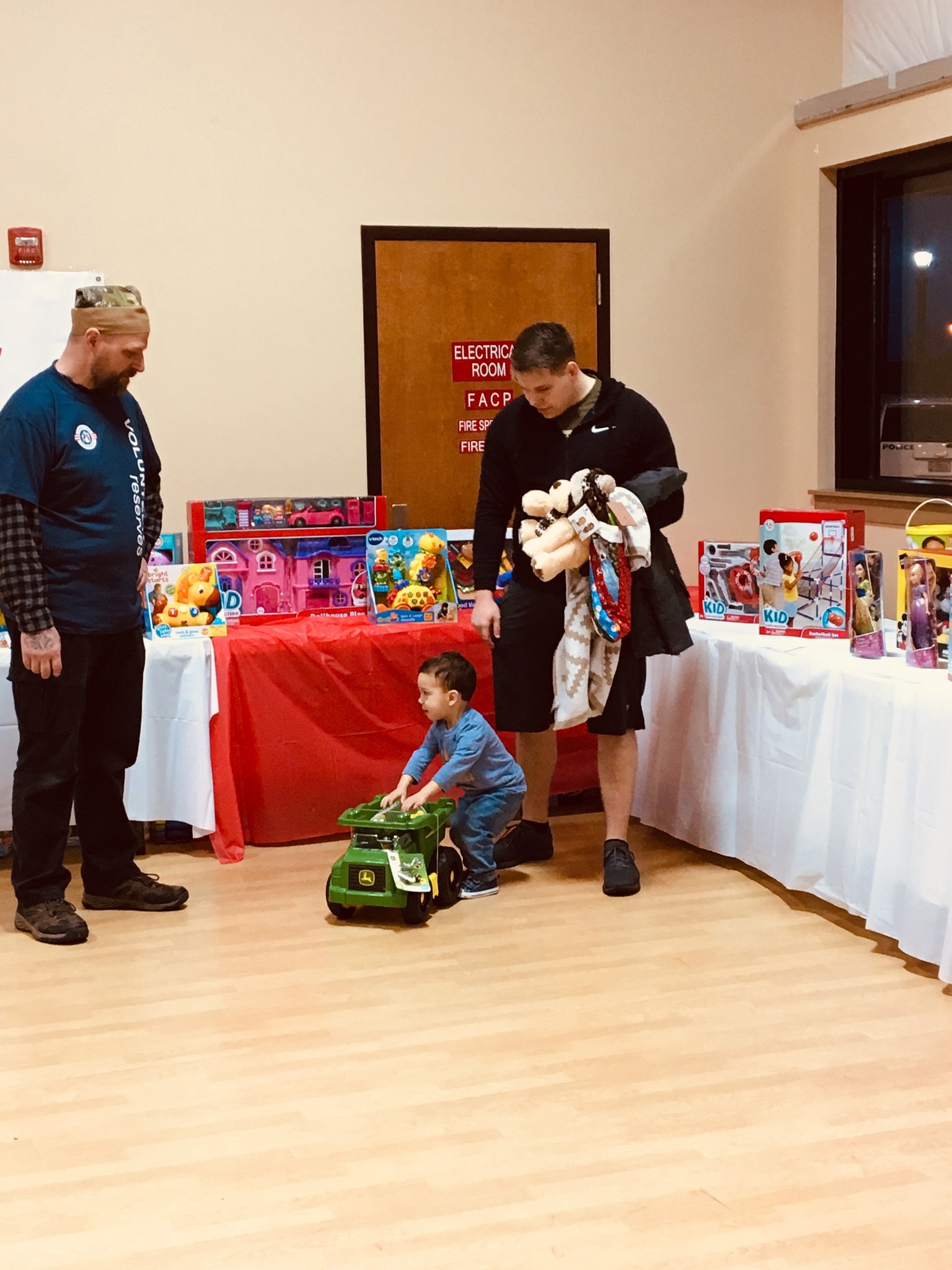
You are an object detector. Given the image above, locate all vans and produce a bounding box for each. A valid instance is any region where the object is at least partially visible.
[879,394,952,498]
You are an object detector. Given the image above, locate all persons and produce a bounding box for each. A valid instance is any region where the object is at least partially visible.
[148,583,162,606]
[470,321,695,897]
[908,564,932,649]
[854,559,873,632]
[0,285,190,945]
[760,539,804,628]
[380,651,527,898]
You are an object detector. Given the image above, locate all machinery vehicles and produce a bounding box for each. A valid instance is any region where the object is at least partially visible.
[325,792,466,925]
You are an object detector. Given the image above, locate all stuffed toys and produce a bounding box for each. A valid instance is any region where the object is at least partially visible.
[518,469,616,583]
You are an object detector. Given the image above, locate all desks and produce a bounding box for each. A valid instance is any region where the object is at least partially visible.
[0,635,219,854]
[208,608,599,864]
[629,612,951,990]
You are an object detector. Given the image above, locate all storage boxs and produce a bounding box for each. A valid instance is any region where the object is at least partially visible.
[365,530,459,626]
[143,563,228,641]
[698,541,759,623]
[759,508,865,639]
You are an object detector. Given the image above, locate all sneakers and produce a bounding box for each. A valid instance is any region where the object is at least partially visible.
[492,819,554,869]
[603,839,640,897]
[82,870,189,912]
[14,898,89,945]
[457,871,500,899]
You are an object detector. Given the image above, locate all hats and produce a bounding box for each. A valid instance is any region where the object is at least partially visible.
[71,285,150,335]
